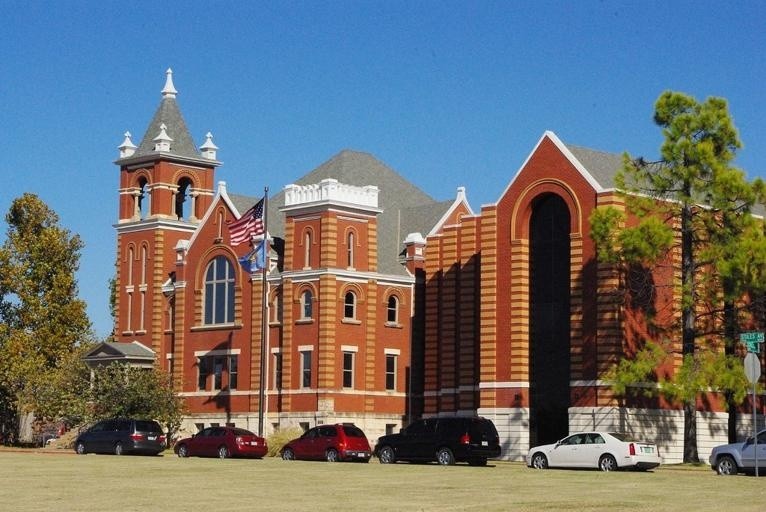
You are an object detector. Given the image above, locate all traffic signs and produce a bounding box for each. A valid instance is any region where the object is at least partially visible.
[739,332,765,342]
[747,341,761,353]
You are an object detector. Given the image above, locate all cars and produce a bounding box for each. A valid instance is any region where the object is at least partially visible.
[524,431,661,472]
[708,428,766,476]
[280,424,372,464]
[173,427,269,459]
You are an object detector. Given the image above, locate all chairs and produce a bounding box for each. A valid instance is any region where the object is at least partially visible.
[580,438,585,443]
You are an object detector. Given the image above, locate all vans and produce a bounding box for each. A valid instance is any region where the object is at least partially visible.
[73,417,167,456]
[373,416,502,466]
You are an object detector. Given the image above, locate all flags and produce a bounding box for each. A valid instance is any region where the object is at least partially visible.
[238,240,267,273]
[226,198,266,246]
